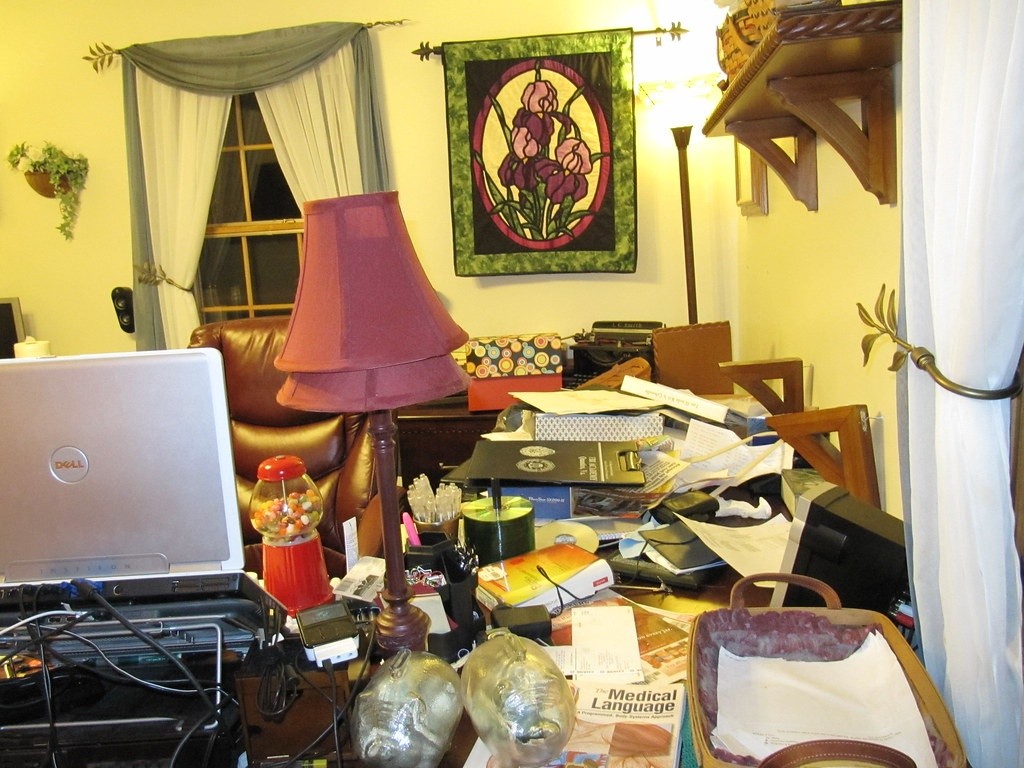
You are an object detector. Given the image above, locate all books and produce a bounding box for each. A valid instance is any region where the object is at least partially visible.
[537,681,686,768]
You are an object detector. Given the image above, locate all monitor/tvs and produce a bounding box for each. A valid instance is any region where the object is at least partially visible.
[0,297,26,361]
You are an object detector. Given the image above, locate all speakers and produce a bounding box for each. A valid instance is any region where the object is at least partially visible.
[112,287,135,334]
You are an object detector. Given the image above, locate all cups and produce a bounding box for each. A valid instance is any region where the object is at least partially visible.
[412,512,463,547]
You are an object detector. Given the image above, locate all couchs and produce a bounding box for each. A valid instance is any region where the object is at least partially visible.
[188,315,378,555]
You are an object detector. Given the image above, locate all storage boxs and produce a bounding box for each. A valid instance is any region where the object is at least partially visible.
[653,320,734,395]
[465,333,562,379]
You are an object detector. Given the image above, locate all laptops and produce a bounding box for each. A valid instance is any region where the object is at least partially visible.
[0,346,301,639]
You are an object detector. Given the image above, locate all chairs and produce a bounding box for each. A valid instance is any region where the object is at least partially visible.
[241,544,351,581]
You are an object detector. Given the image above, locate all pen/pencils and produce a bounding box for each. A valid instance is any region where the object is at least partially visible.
[404,471,465,524]
[401,513,421,548]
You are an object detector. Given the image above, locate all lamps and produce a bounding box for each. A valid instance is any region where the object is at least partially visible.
[273,193,470,647]
[651,90,711,324]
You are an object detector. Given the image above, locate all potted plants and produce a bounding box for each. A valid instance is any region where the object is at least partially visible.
[4,141,89,241]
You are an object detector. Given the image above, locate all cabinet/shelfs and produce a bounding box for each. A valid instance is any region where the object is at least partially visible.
[397,399,502,524]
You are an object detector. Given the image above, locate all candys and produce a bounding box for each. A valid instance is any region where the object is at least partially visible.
[250,489,320,541]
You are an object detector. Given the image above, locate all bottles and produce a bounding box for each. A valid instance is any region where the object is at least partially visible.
[248,454,336,617]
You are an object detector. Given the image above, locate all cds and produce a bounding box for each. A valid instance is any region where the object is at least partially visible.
[462,496,535,567]
[535,521,598,555]
